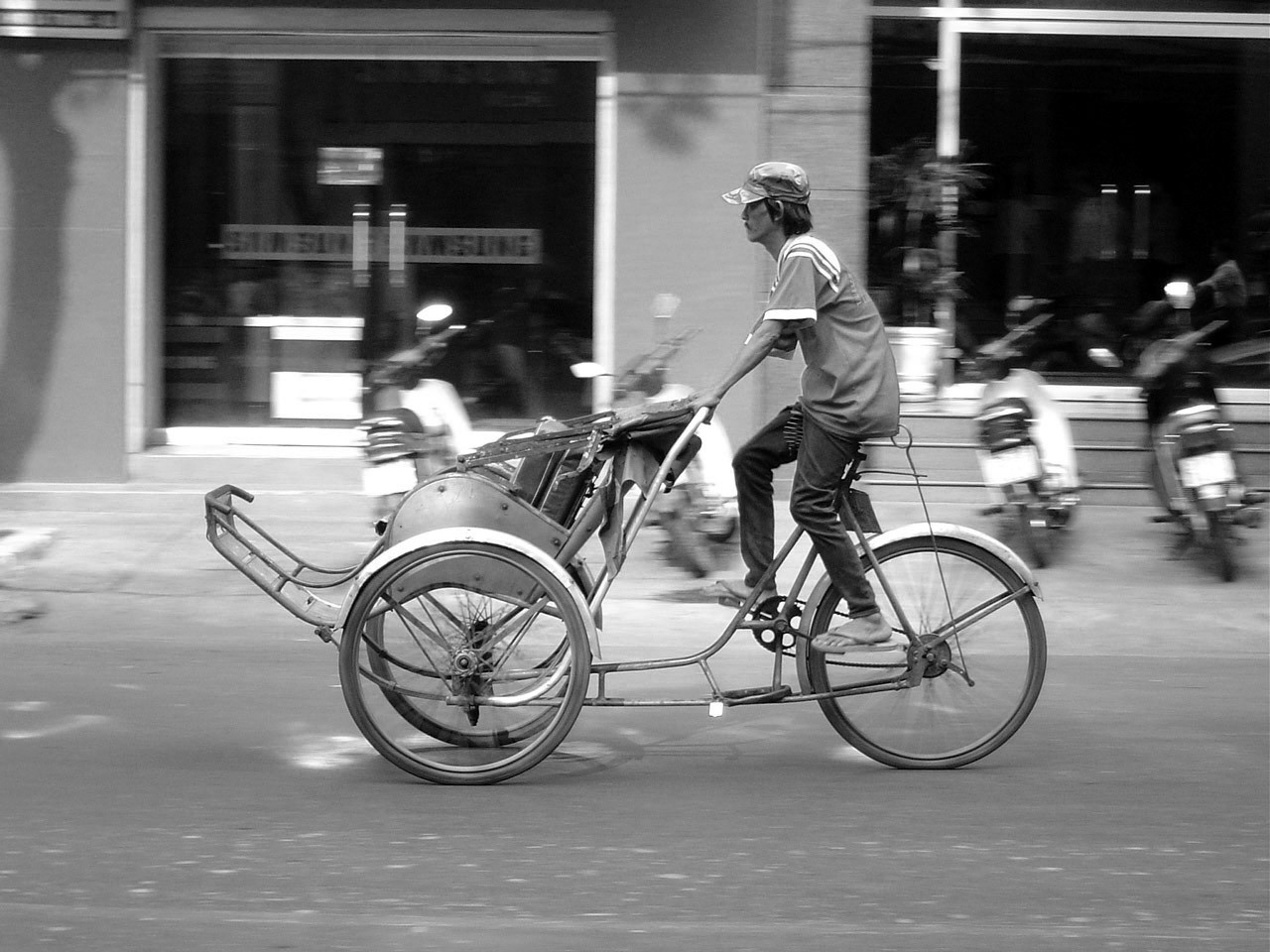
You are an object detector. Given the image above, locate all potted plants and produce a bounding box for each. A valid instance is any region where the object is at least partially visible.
[857,135,994,404]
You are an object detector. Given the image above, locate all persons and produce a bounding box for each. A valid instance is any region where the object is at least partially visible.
[683,160,903,651]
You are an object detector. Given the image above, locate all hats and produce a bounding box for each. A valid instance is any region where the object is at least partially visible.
[721,159,810,205]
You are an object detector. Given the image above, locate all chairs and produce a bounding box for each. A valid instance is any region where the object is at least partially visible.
[453,415,596,529]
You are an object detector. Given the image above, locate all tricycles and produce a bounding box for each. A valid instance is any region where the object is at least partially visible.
[206,405,1049,785]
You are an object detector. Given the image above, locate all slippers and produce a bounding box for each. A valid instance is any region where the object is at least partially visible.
[700,577,764,609]
[809,626,900,652]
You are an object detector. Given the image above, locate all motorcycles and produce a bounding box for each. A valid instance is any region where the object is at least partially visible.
[355,301,477,539]
[1087,260,1268,583]
[971,296,1082,566]
[568,293,738,578]
